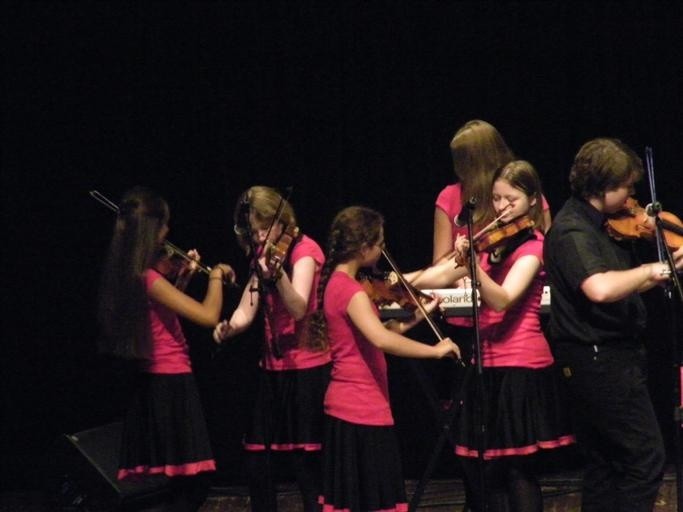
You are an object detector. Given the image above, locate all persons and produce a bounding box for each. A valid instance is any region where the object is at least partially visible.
[434,120,552,357]
[114,185,235,510]
[542,136,682,511]
[454,161,576,511]
[307,209,461,510]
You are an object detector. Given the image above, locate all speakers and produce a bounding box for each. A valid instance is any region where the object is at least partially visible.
[46,423,173,511]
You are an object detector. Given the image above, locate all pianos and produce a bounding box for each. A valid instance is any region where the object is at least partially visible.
[375,286,553,316]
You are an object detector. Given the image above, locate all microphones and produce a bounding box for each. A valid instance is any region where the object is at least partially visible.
[232,199,255,235]
[454,197,478,226]
[646,202,661,217]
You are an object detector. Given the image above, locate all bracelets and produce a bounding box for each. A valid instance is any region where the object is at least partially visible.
[208,276,223,281]
[212,265,224,276]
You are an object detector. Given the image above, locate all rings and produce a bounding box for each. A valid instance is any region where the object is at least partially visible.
[660,271,664,276]
[663,266,666,271]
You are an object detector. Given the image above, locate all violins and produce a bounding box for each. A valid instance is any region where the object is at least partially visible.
[356,265,442,313]
[145,251,210,278]
[474,215,536,253]
[604,196,681,253]
[265,223,300,278]
